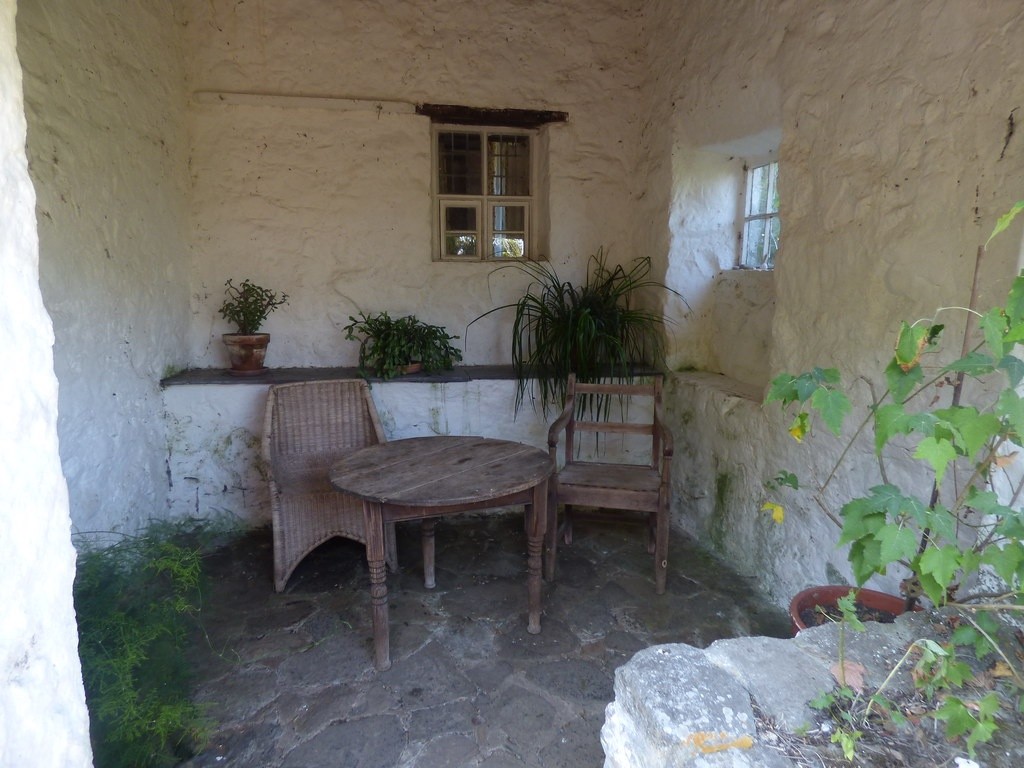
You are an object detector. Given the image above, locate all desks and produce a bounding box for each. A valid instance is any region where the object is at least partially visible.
[328,436,556,673]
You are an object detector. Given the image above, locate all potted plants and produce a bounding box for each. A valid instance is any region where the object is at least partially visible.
[217,277,291,371]
[340,310,463,384]
[461,241,696,460]
[756,199,1024,761]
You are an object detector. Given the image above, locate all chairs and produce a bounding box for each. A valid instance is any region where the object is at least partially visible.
[258,380,400,591]
[547,373,674,595]
